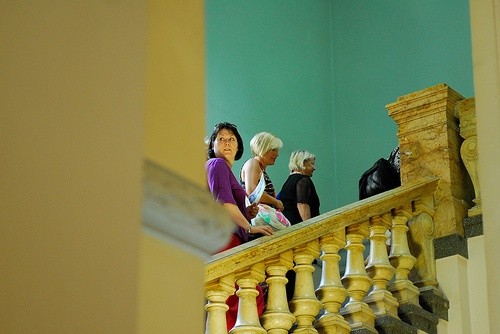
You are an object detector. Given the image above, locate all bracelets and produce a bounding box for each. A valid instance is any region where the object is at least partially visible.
[246,224,251,234]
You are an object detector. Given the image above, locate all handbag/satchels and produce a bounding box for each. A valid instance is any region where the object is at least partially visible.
[251,203,291,232]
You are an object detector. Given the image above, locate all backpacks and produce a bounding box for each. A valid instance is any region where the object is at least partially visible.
[359,147,401,200]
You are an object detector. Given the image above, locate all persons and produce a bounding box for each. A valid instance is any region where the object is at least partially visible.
[385,143,402,173]
[205,121,266,332]
[277,150,320,310]
[239,131,284,309]
